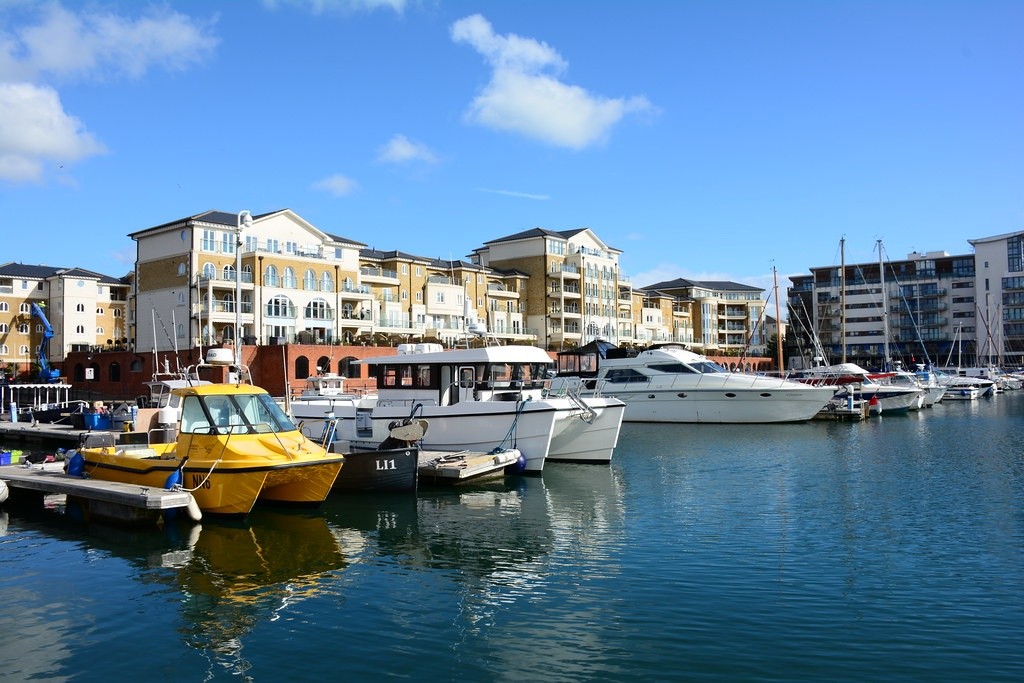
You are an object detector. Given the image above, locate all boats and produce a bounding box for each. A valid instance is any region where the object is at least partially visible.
[544,338,840,426]
[0,383,90,426]
[276,252,627,497]
[63,276,346,521]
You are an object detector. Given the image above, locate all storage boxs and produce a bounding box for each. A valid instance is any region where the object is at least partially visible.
[83,413,112,430]
[0,450,24,466]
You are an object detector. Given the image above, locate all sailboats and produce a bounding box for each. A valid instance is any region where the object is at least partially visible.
[734,234,1024,416]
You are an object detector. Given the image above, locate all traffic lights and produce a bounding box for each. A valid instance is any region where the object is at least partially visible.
[5,373,14,385]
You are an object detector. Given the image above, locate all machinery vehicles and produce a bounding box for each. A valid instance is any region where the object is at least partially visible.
[27,301,60,384]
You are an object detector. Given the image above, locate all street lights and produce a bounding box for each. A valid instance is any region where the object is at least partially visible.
[236,210,253,370]
